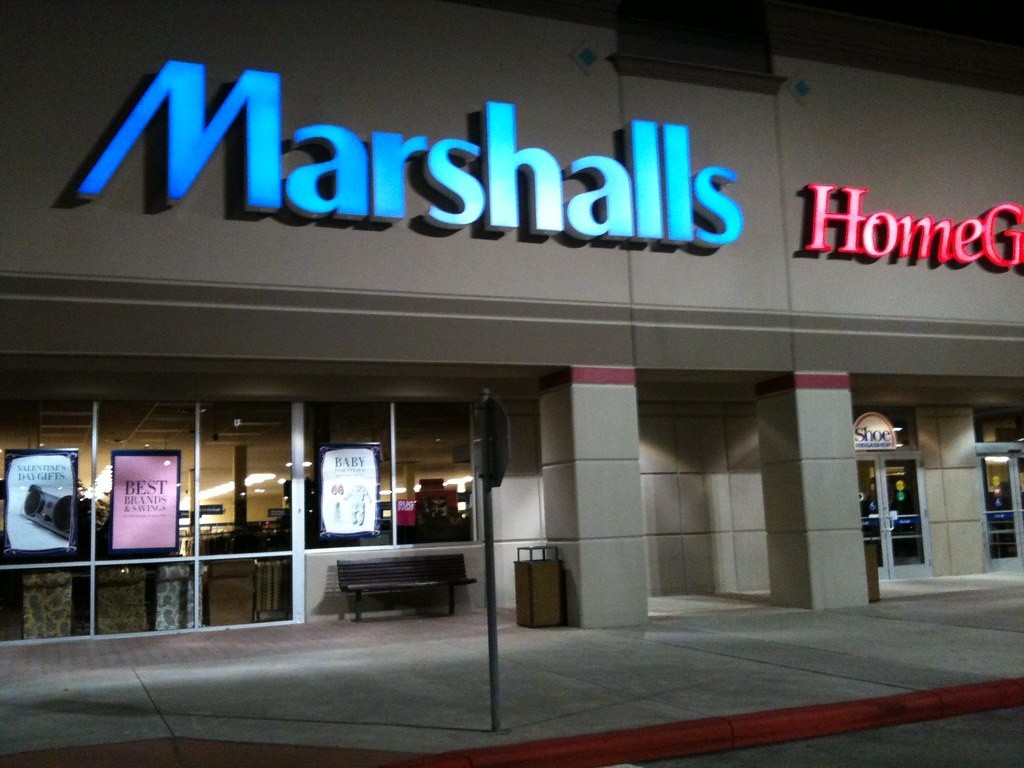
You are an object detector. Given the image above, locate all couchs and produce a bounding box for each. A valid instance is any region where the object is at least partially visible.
[252,558,292,623]
[154,560,210,630]
[95,566,151,635]
[20,570,76,639]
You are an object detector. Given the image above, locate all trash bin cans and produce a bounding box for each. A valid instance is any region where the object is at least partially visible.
[512,545,565,627]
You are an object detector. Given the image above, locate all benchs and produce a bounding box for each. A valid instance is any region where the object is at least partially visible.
[336,553,477,623]
